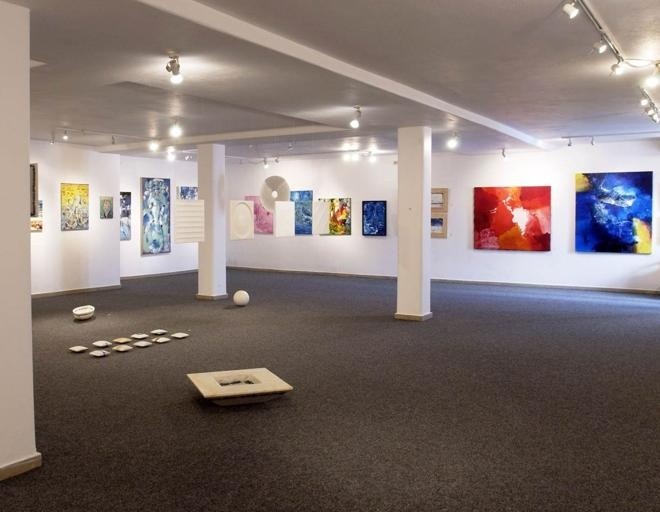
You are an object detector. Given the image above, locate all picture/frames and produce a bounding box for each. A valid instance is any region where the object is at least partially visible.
[431,213,448,238]
[431,188,448,212]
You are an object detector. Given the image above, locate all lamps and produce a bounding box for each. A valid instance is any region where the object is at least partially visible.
[179,149,280,167]
[638,81,660,125]
[560,0,629,80]
[349,104,363,129]
[46,127,162,152]
[164,48,184,85]
